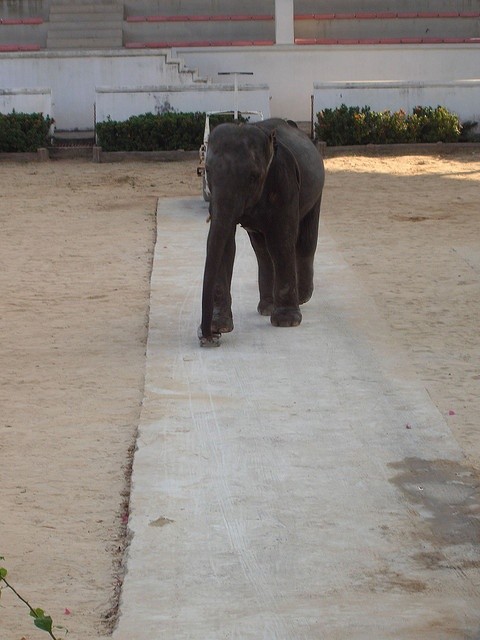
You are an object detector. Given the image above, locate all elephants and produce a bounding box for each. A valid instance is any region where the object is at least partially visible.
[196,115,326,348]
